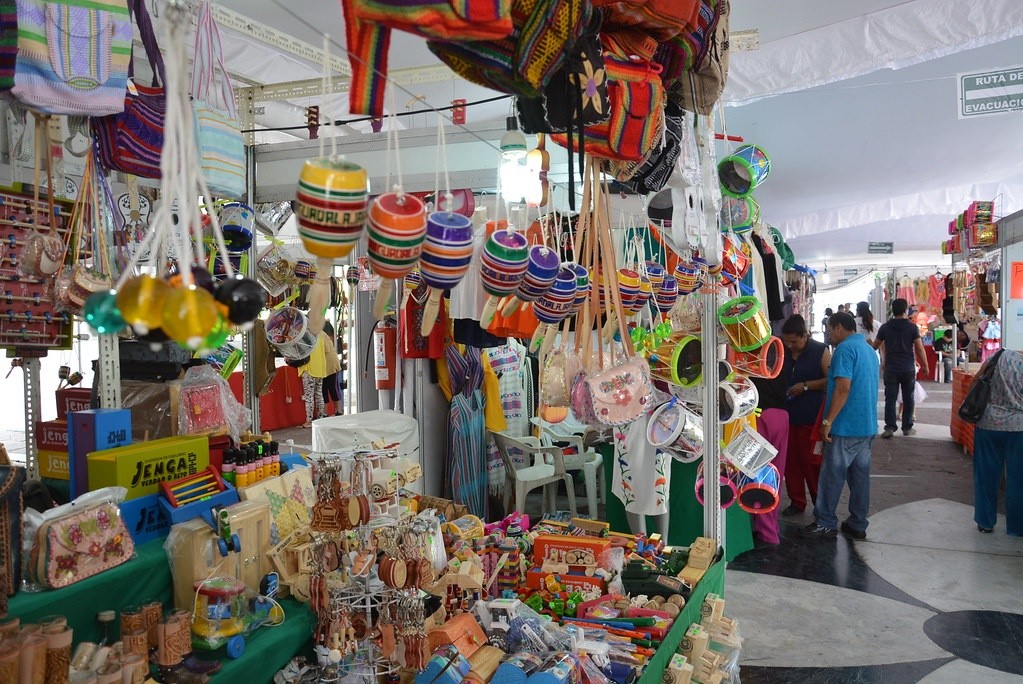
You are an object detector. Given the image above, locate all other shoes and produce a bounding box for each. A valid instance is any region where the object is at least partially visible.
[977,524,993,533]
[783,506,795,516]
[880,429,894,438]
[903,428,917,436]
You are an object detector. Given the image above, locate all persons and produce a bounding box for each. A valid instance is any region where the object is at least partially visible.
[933,329,962,382]
[798,312,880,538]
[968,348,1023,536]
[749,314,832,547]
[298,320,342,428]
[822,298,929,437]
[611,412,672,546]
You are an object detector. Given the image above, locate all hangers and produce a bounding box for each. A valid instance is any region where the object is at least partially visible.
[904,267,944,279]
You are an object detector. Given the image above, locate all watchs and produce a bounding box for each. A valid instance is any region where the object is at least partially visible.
[804,381,808,390]
[823,419,830,425]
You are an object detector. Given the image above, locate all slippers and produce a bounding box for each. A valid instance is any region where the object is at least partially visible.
[296,424,310,428]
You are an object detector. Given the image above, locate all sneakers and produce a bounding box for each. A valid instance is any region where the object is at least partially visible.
[841,520,867,539]
[798,522,838,537]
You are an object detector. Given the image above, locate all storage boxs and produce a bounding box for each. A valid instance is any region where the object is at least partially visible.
[35,340,235,547]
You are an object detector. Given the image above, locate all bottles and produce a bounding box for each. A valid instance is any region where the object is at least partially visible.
[785,367,796,400]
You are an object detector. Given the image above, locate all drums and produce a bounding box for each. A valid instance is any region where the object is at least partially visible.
[217,202,292,253]
[647,142,782,512]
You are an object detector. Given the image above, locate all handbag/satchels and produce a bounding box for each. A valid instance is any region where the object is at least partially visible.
[189,0,247,198]
[91,0,168,179]
[340,0,729,197]
[10,0,127,117]
[34,502,134,588]
[544,348,651,425]
[17,222,114,319]
[957,347,1005,424]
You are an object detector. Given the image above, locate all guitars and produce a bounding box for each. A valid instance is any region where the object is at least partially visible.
[434,98,478,218]
[524,131,550,207]
[116,171,158,268]
[291,104,321,212]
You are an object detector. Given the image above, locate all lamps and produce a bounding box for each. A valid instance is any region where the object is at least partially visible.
[820,254,833,285]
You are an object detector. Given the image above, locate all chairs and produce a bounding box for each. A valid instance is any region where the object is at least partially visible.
[483,430,580,522]
[528,416,609,521]
[933,347,965,383]
